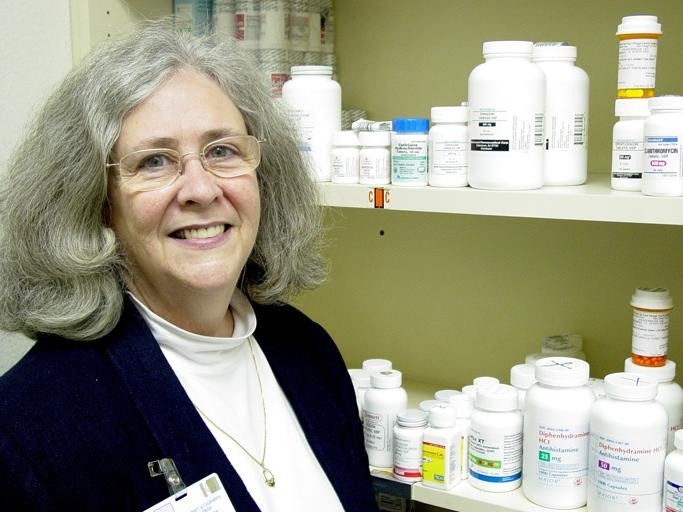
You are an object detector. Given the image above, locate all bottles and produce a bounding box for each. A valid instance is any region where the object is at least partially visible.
[347,287,683,511]
[612,14,683,199]
[278,39,591,191]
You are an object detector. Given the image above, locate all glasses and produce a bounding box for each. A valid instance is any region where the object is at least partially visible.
[101,133,275,192]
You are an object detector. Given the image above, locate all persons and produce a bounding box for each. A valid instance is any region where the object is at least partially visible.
[2,14,378,510]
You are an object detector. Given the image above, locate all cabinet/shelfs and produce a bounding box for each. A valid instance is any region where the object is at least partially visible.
[295,181,683,512]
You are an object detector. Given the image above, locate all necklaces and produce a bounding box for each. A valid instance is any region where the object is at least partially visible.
[194,336,275,486]
